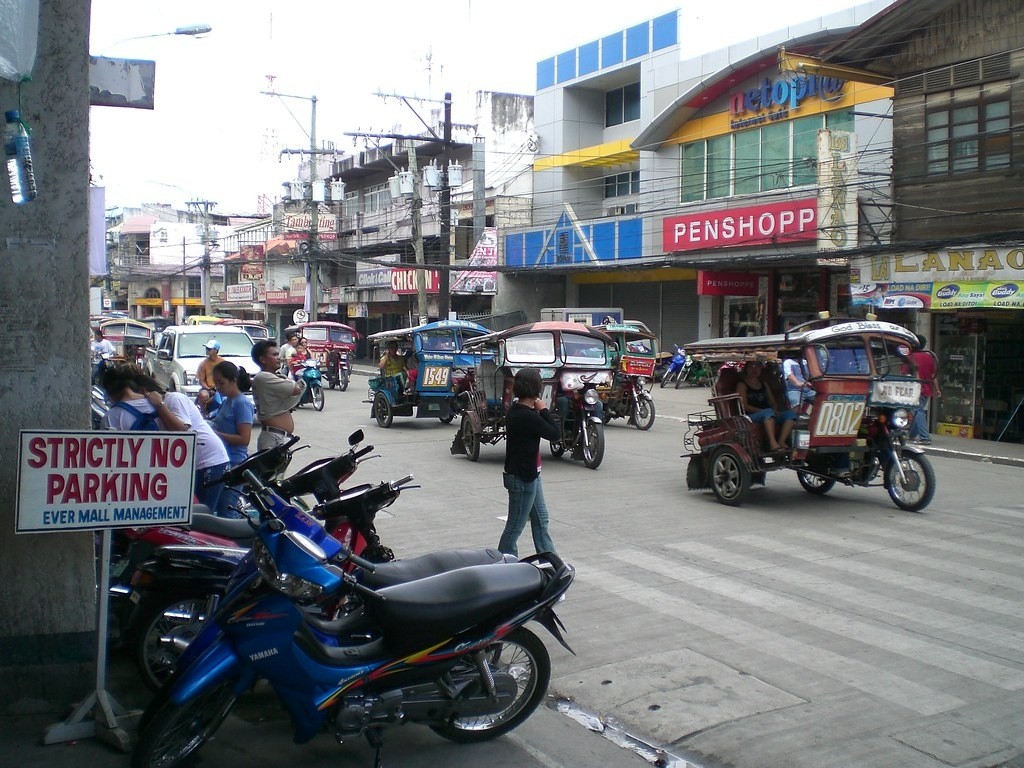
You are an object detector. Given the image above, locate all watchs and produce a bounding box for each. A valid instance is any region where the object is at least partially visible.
[156,402,165,408]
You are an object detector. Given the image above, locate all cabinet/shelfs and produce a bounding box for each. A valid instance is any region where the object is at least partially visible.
[936,332,1024,439]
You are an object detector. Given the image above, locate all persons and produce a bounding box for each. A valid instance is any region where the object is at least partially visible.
[498,366,566,602]
[734,357,817,451]
[279,332,312,383]
[379,341,418,399]
[90,328,253,519]
[900,335,941,445]
[555,345,603,437]
[249,339,307,482]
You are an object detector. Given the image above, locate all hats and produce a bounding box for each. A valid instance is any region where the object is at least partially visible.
[202,340,220,351]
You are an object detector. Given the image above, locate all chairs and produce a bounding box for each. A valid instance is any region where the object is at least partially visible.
[715,362,785,442]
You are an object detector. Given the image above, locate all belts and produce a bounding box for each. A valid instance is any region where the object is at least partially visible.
[262,427,292,438]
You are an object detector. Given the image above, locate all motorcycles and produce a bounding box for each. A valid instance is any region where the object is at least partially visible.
[449,321,617,469]
[96,429,576,768]
[283,322,360,391]
[286,353,326,411]
[680,316,940,511]
[90,317,153,366]
[580,323,662,431]
[187,315,277,346]
[363,320,496,429]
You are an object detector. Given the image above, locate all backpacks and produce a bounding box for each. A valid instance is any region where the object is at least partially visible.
[111,402,158,431]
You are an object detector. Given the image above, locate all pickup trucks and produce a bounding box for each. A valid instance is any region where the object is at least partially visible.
[142,324,261,410]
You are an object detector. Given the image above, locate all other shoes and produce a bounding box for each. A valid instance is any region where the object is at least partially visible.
[914,439,931,444]
[770,446,792,452]
